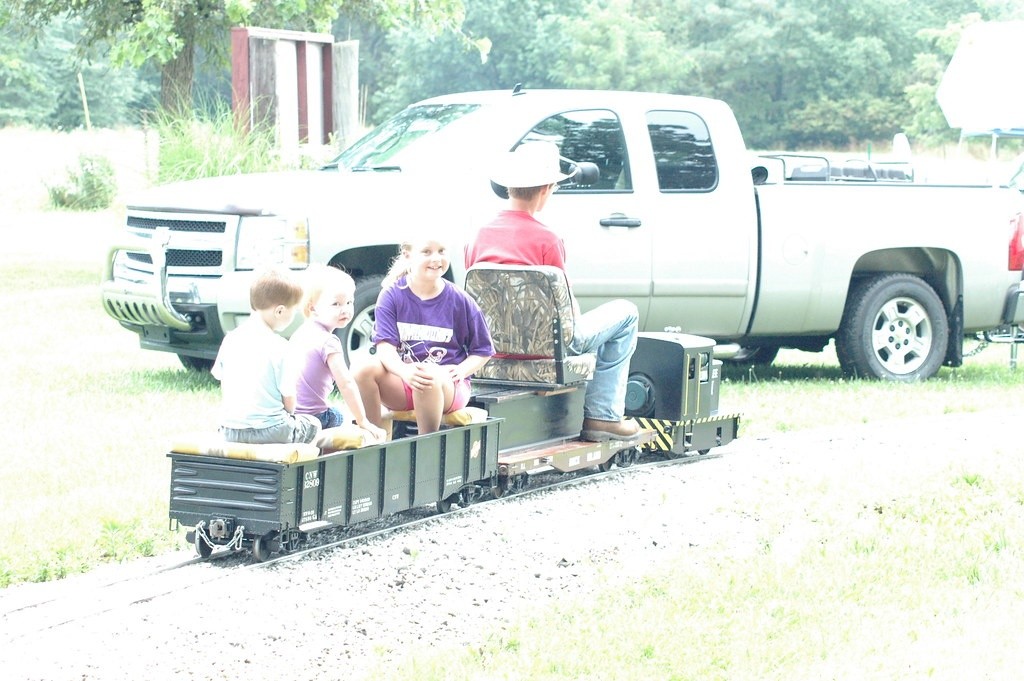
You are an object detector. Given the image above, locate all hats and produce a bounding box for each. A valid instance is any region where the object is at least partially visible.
[486,136,581,189]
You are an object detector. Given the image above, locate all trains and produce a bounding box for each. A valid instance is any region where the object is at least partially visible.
[165,258,742,565]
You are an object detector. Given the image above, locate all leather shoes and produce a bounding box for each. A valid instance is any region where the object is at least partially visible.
[579,408,639,444]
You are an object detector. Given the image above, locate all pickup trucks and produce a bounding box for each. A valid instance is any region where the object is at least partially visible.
[100,83,1023,384]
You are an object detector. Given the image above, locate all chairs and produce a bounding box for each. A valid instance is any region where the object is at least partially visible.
[460,261,597,388]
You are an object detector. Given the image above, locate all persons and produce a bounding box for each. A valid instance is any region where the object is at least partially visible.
[348,225,496,435]
[288,266,389,443]
[464,140,641,443]
[210,268,322,446]
